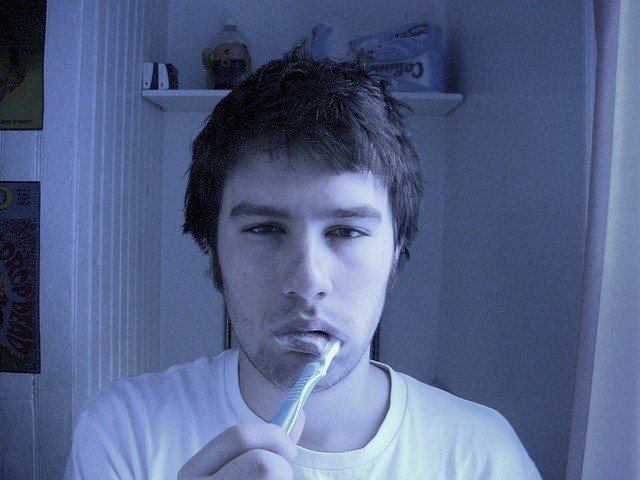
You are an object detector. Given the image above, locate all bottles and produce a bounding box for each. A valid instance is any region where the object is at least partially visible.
[202,17,251,90]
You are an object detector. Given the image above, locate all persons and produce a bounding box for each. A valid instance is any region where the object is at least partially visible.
[65,38,543,478]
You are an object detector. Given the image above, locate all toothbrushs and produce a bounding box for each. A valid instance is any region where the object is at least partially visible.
[269,337,341,435]
